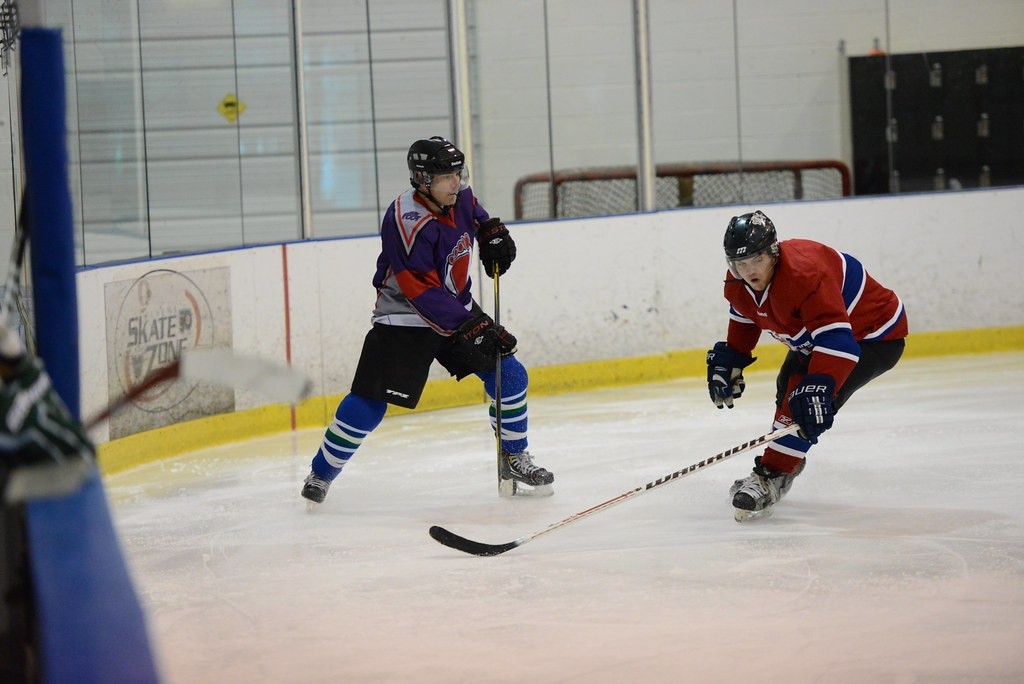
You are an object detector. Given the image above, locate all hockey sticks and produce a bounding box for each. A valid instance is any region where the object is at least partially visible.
[80,347,314,436]
[0,183,32,331]
[492,257,555,501]
[428,422,802,558]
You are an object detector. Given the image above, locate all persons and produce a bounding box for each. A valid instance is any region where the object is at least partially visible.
[302,136,555,511]
[706,211,908,524]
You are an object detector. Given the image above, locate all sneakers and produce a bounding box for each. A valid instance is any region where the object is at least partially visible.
[731,473,758,496]
[732,456,806,525]
[499,451,555,497]
[301,471,332,511]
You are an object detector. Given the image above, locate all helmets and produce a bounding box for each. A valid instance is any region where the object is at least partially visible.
[407,136,465,188]
[724,210,776,262]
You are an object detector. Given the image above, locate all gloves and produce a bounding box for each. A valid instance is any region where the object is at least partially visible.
[789,374,835,444]
[475,217,517,278]
[707,342,757,408]
[457,313,517,361]
